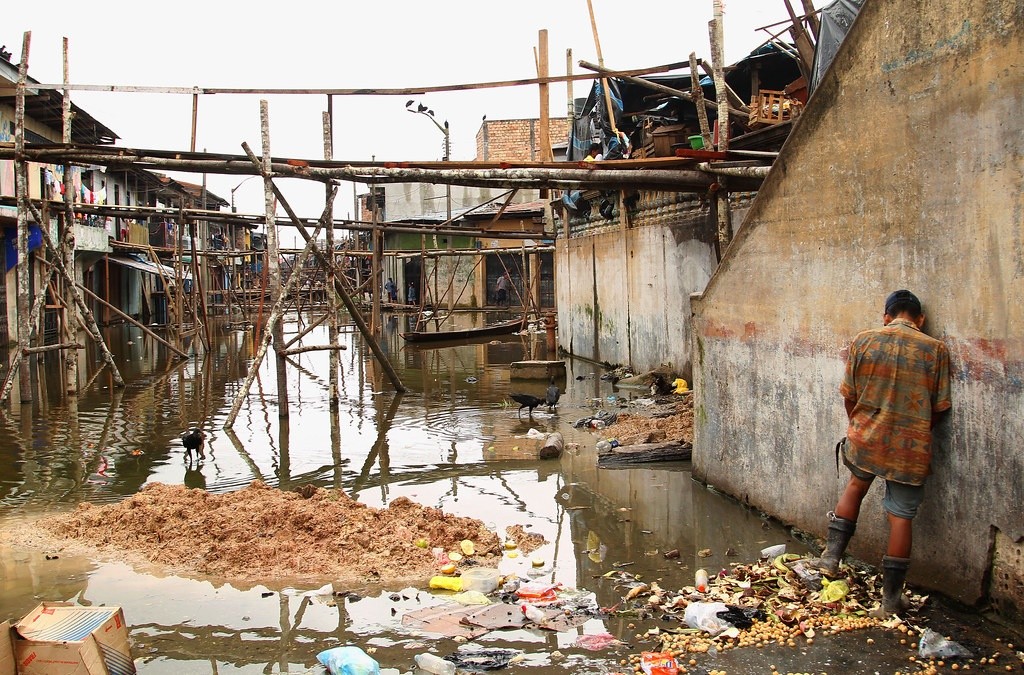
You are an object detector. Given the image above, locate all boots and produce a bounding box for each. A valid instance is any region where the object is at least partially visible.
[869,554,910,621]
[807,516,855,577]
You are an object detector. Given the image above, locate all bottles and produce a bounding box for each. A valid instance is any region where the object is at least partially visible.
[695,569,708,593]
[414,652,456,675]
[596,438,623,453]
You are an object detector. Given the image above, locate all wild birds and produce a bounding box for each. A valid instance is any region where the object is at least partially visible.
[546,375,560,409]
[508,394,548,418]
[182,428,203,461]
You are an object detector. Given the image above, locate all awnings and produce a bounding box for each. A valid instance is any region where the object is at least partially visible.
[382,200,546,225]
[0,57,267,281]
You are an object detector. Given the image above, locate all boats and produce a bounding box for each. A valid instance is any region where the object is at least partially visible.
[397,314,530,342]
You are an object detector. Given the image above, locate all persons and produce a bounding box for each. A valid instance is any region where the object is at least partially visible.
[407,281,417,305]
[383,277,400,305]
[812,289,952,616]
[496,272,509,306]
[582,142,603,161]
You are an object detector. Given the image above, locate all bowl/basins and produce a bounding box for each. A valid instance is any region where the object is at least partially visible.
[461,567,500,593]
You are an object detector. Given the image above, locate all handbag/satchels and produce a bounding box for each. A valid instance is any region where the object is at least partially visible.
[594,409,616,426]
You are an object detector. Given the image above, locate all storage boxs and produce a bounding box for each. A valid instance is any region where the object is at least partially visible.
[0,601,137,675]
[653,123,686,155]
